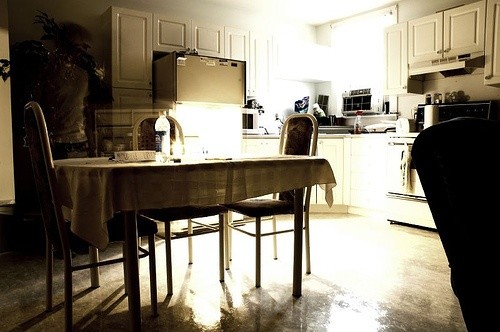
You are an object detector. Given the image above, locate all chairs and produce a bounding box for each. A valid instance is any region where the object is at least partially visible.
[224,114,321,288]
[132,116,227,295]
[23,99,159,331]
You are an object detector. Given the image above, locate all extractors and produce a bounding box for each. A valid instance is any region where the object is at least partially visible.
[440,65,473,76]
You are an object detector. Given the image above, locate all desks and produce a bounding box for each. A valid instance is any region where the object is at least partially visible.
[50,149,329,328]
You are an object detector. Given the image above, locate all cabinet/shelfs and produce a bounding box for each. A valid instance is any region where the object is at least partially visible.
[110,6,152,89]
[484,0,500,84]
[225,28,277,97]
[154,14,224,58]
[409,1,487,64]
[381,21,408,94]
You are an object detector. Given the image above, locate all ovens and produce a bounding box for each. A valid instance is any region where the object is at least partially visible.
[387,137,426,201]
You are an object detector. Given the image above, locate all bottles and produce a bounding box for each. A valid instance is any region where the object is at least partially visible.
[353,112,362,134]
[154,110,170,161]
